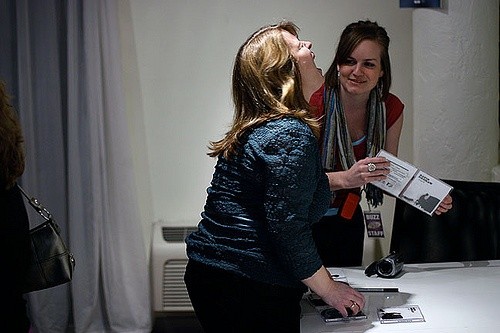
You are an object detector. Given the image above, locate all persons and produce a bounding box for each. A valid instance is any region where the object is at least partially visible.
[184,18,366,333]
[308,20,452,268]
[0,80,32,333]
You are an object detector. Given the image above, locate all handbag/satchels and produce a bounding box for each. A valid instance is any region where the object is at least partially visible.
[15,182,74,294]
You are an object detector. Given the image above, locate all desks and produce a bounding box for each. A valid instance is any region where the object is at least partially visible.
[300,259,500,333]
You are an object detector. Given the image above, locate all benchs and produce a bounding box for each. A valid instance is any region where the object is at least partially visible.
[390,180,500,262]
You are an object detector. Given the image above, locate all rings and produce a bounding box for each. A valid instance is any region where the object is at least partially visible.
[367,163,376,172]
[350,303,356,309]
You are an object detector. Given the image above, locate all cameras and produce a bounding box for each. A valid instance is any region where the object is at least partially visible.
[376,250,405,278]
